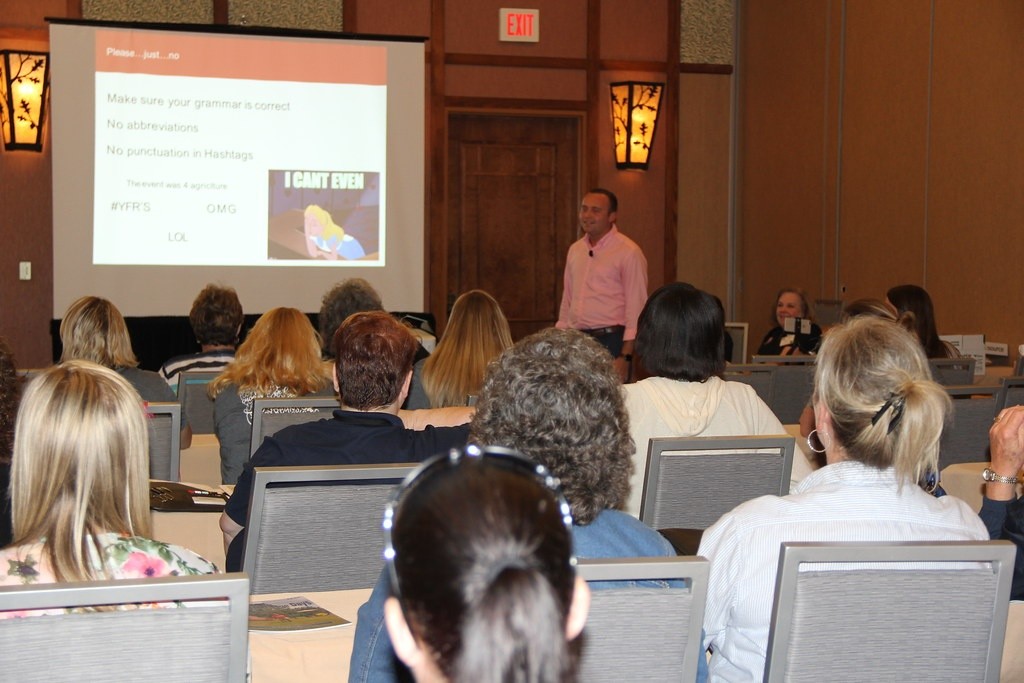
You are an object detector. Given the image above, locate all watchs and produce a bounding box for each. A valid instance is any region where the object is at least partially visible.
[982,467,1018,484]
[617,353,633,362]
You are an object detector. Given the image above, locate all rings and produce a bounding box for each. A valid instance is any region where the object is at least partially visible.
[992,417,1001,423]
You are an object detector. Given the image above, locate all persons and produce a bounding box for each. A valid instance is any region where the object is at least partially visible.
[553,187,649,386]
[692,315,989,683]
[382,445,592,683]
[346,325,710,683]
[0,359,230,618]
[53,278,1024,601]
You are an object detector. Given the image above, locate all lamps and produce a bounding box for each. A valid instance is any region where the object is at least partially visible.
[609,81,668,170]
[0,49,51,152]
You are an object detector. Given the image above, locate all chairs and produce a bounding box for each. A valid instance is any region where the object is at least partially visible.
[639,436,797,533]
[144,370,341,484]
[761,540,1016,683]
[570,557,709,683]
[241,463,426,594]
[722,355,1024,467]
[0,573,251,683]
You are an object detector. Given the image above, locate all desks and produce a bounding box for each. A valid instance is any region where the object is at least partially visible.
[149,479,236,572]
[247,589,382,683]
[939,460,1024,521]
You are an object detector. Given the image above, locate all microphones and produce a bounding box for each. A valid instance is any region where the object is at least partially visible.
[589,250,593,257]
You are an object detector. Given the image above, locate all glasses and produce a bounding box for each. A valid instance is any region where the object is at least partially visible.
[384,445,578,599]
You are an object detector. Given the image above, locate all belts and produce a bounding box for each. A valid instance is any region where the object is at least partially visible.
[580,326,625,337]
